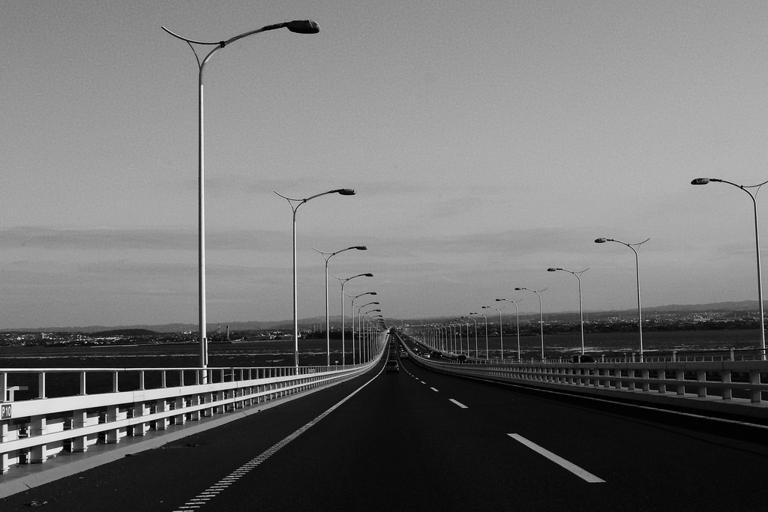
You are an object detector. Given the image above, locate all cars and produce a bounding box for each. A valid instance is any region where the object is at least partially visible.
[387,336,430,372]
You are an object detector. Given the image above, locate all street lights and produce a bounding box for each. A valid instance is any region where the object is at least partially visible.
[276,190,356,373]
[334,273,387,369]
[595,237,645,362]
[160,18,320,386]
[425,305,506,361]
[313,246,367,365]
[514,287,544,361]
[546,268,588,361]
[496,297,523,363]
[692,178,768,362]
